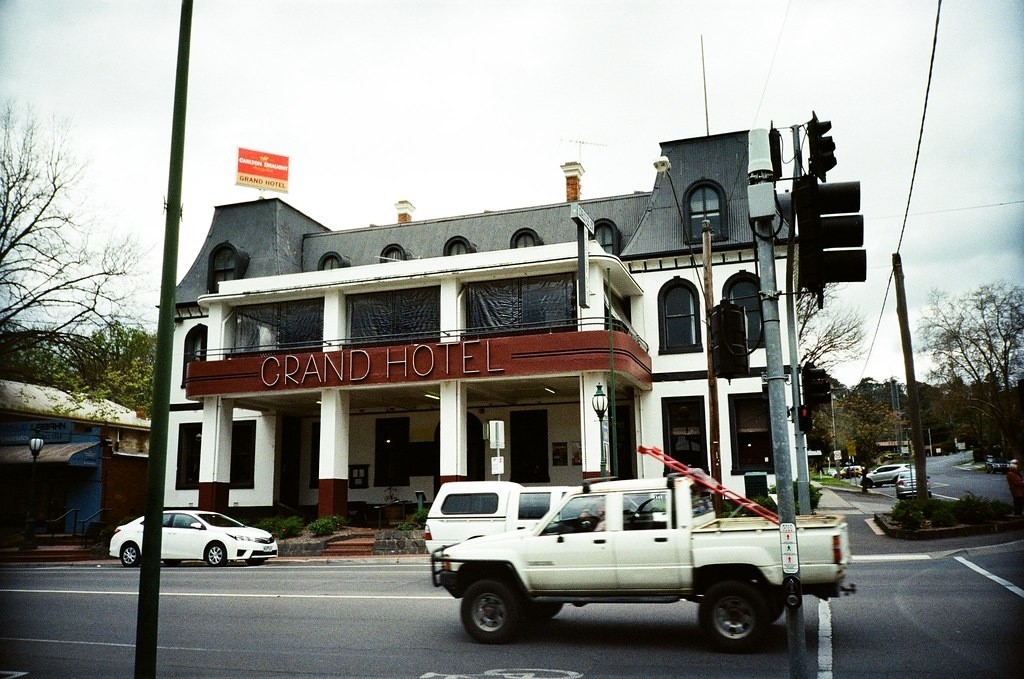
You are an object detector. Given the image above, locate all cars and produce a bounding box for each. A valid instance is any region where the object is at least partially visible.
[985,455,1012,474]
[108,510,278,567]
[894,471,932,500]
[860,464,914,489]
[836,466,864,480]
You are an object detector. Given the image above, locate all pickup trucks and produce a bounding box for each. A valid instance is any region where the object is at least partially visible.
[422,481,642,562]
[429,470,852,655]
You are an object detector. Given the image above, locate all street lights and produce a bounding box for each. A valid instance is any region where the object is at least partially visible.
[18,427,45,550]
[593,381,609,477]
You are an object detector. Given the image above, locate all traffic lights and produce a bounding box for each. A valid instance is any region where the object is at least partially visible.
[795,172,867,288]
[807,112,840,184]
[801,361,832,410]
[799,405,814,432]
